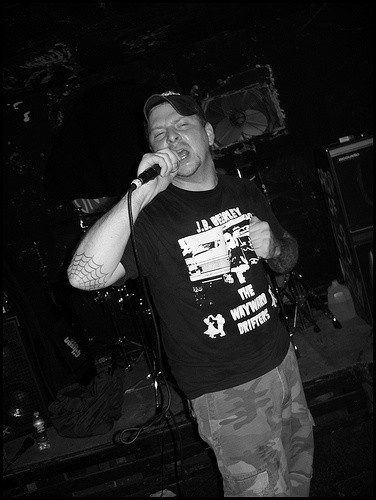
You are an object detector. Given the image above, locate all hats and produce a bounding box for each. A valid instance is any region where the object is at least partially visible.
[144,91,207,124]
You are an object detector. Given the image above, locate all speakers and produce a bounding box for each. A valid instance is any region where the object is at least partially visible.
[2,313,53,442]
[319,129,376,321]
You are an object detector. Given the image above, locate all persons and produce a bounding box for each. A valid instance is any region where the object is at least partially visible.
[66,91,316,497]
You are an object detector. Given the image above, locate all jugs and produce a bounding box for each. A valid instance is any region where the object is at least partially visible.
[327,280,355,323]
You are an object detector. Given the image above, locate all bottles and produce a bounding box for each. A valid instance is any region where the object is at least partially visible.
[32,412,47,449]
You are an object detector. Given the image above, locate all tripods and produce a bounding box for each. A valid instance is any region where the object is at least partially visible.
[251,166,341,332]
[93,289,153,375]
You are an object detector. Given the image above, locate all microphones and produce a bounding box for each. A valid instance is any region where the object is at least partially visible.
[8,437,35,467]
[129,164,161,190]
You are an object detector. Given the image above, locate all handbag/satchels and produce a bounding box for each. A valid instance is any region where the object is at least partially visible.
[47,383,124,438]
